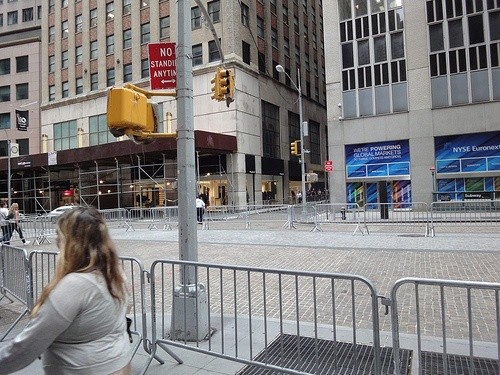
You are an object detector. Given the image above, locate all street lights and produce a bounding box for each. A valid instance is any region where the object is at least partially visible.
[275,64,307,221]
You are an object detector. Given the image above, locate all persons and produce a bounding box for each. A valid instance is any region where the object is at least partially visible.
[196,196,206,225]
[0,204,133,375]
[0,202,30,245]
[290,191,297,205]
[306,188,329,204]
[296,191,302,204]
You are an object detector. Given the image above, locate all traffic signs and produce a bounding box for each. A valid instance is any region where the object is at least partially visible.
[147,42,177,91]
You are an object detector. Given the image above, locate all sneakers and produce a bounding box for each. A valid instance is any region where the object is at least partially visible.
[23,241,31,246]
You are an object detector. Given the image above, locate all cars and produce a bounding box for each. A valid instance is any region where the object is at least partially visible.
[48,206,80,223]
[7,208,24,220]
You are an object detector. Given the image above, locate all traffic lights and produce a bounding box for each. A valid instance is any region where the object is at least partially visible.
[217,67,230,100]
[211,74,218,100]
[291,140,301,156]
